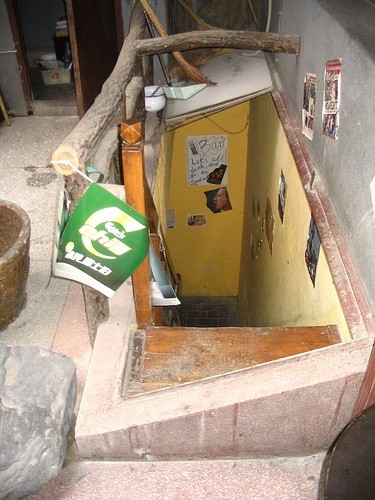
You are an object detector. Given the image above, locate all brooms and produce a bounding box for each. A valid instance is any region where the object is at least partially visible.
[138,0,234,86]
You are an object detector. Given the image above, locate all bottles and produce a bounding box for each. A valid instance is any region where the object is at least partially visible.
[53,20,71,64]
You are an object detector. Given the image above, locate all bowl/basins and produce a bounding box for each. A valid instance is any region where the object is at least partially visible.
[39,54,57,69]
[145,86,166,113]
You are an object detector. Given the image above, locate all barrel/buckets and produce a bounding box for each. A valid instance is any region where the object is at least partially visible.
[0,199,32,331]
